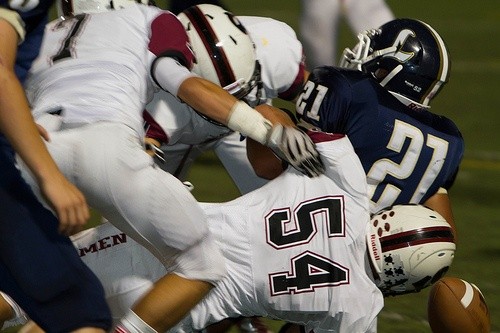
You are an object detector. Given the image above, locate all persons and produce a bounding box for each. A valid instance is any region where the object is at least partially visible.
[0,128,456,333]
[299,0,395,71]
[207,18,464,333]
[0,0,325,333]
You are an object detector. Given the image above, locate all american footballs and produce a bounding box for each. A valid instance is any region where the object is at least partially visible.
[426,275,492,333]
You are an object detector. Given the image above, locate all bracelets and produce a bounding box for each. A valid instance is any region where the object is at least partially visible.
[226,100,272,146]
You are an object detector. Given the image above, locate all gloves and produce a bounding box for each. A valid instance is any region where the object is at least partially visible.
[266,124,326,178]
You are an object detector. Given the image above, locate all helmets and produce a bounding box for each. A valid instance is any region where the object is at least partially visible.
[362,17,448,109]
[176,4,260,108]
[55,0,152,18]
[365,203,456,297]
